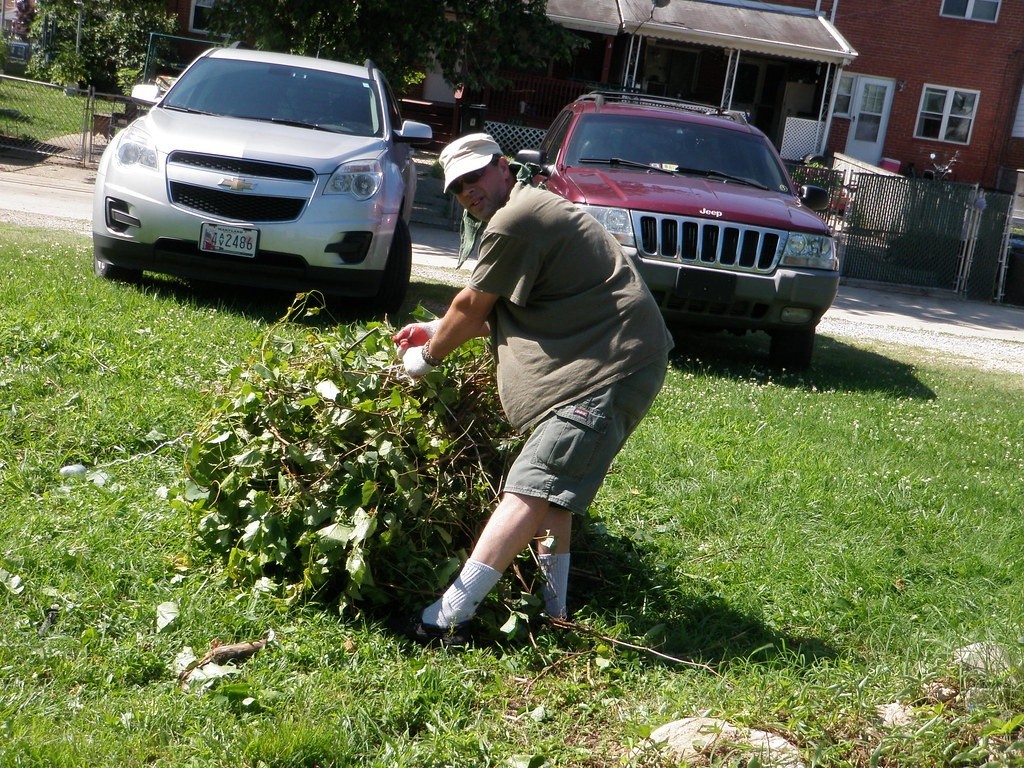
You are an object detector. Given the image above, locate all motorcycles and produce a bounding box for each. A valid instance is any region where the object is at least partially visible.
[922,148,963,185]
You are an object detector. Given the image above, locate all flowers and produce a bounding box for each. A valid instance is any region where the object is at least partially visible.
[791,164,840,194]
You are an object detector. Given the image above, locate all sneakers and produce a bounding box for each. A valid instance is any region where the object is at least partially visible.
[391,607,480,648]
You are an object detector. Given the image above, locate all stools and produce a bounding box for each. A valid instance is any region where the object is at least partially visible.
[93,113,115,139]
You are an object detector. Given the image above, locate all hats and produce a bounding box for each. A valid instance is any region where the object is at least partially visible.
[437,132,503,192]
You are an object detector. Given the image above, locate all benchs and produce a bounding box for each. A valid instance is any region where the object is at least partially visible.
[398,100,461,146]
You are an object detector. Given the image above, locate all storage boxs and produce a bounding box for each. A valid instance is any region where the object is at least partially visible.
[880,157,901,175]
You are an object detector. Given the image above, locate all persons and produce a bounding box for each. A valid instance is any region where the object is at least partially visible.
[392,133,675,659]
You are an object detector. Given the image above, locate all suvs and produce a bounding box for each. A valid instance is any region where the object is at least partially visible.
[514,90,841,371]
[91,39,435,318]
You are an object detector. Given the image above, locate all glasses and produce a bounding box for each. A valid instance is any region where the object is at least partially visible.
[449,167,485,193]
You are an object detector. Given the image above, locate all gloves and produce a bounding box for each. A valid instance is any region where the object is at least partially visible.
[403,339,440,377]
[393,316,444,355]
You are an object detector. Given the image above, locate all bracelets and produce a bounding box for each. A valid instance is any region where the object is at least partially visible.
[421,337,443,366]
